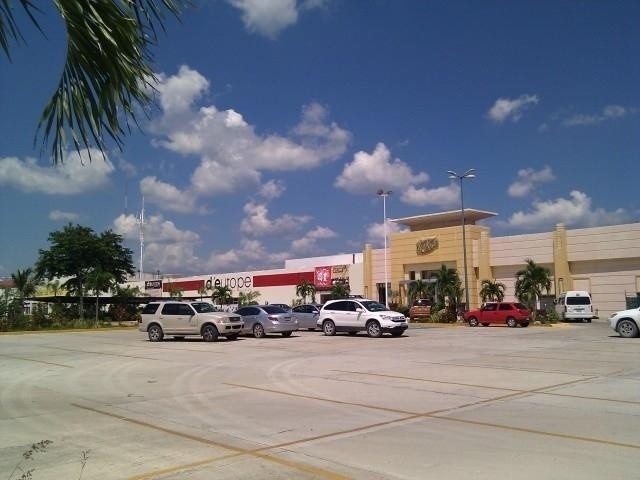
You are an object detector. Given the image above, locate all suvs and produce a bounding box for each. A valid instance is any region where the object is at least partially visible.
[138,301,245,342]
[316,299,409,338]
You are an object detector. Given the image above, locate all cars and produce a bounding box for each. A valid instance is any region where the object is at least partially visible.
[409,298,431,320]
[607,306,640,338]
[462,301,532,328]
[232,303,324,338]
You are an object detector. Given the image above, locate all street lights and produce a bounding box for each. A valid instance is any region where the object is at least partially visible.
[376,188,393,310]
[447,167,477,312]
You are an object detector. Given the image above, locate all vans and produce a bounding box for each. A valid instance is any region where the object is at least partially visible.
[553,291,594,323]
